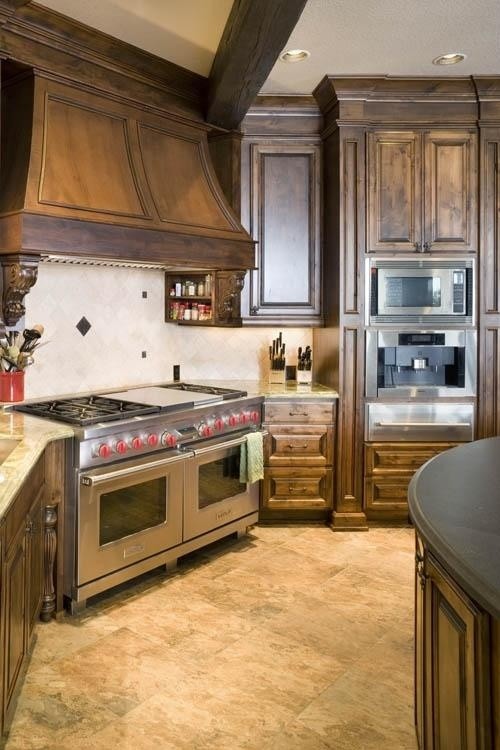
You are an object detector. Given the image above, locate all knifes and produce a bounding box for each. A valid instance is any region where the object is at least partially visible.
[268,331,286,371]
[297,345,312,370]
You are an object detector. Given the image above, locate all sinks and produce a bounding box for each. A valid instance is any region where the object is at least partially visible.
[0,434,25,466]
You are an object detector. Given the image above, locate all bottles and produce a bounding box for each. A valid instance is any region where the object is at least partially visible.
[168,273,213,321]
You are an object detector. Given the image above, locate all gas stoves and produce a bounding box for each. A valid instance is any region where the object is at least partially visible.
[14,377,266,459]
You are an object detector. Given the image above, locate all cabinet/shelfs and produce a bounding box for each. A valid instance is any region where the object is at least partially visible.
[314,74,478,254]
[205,97,328,327]
[346,437,466,520]
[260,399,339,524]
[164,267,247,328]
[414,526,500,750]
[0,451,46,749]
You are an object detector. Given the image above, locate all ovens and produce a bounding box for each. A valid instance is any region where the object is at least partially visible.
[65,426,268,602]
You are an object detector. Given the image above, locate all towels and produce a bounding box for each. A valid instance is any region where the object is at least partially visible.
[238,431,264,483]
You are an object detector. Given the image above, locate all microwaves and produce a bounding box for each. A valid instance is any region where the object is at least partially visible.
[363,253,478,326]
